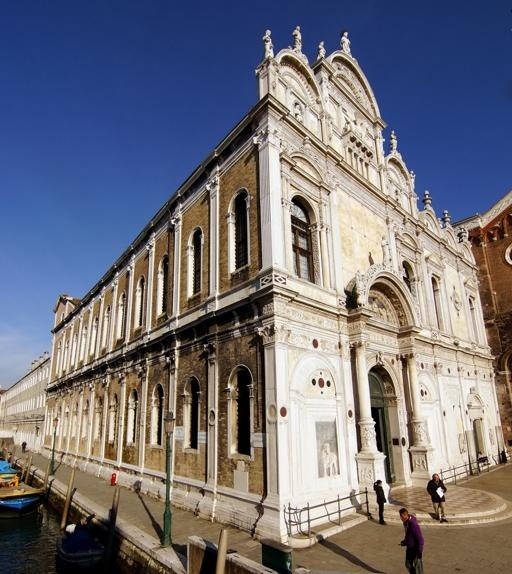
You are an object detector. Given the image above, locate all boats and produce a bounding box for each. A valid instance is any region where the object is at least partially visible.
[0,460,43,512]
[57,531,108,573]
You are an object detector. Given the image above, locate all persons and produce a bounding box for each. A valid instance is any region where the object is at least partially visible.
[410,170,416,189]
[373,480,387,524]
[340,30,351,53]
[317,40,327,61]
[478,451,490,466]
[262,28,275,57]
[292,24,302,53]
[426,473,449,524]
[501,449,508,464]
[399,507,425,573]
[390,129,399,151]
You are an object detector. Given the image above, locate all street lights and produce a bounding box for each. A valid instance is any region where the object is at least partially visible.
[46,416,59,476]
[161,411,177,548]
[110,473,116,487]
